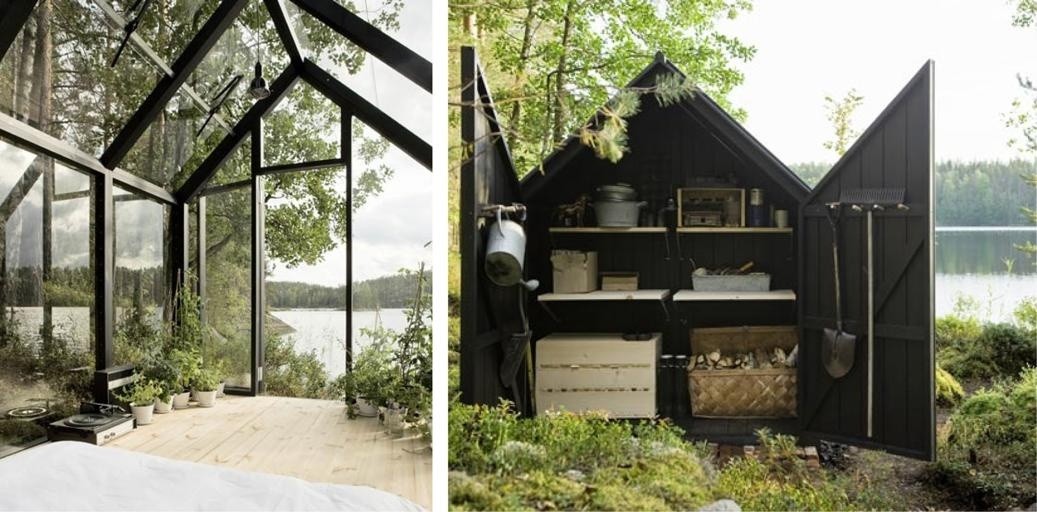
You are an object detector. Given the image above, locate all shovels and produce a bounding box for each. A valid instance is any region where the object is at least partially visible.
[820,201,856,381]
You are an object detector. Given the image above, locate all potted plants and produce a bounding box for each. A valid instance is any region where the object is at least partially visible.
[337,262,432,453]
[111,268,235,426]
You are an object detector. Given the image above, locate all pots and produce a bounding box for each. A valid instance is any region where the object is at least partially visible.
[586,200,647,228]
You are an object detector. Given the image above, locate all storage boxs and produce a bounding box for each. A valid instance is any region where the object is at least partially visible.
[552,247,599,292]
[535,333,664,421]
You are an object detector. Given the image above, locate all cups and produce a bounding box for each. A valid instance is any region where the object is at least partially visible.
[750,188,762,206]
[747,205,769,227]
[775,209,789,229]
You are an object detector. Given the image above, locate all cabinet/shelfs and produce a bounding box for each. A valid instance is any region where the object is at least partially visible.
[537,227,801,322]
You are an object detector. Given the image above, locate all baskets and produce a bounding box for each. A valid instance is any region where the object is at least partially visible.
[688,323,799,418]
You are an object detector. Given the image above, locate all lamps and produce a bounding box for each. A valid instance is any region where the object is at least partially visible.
[247,0,271,100]
